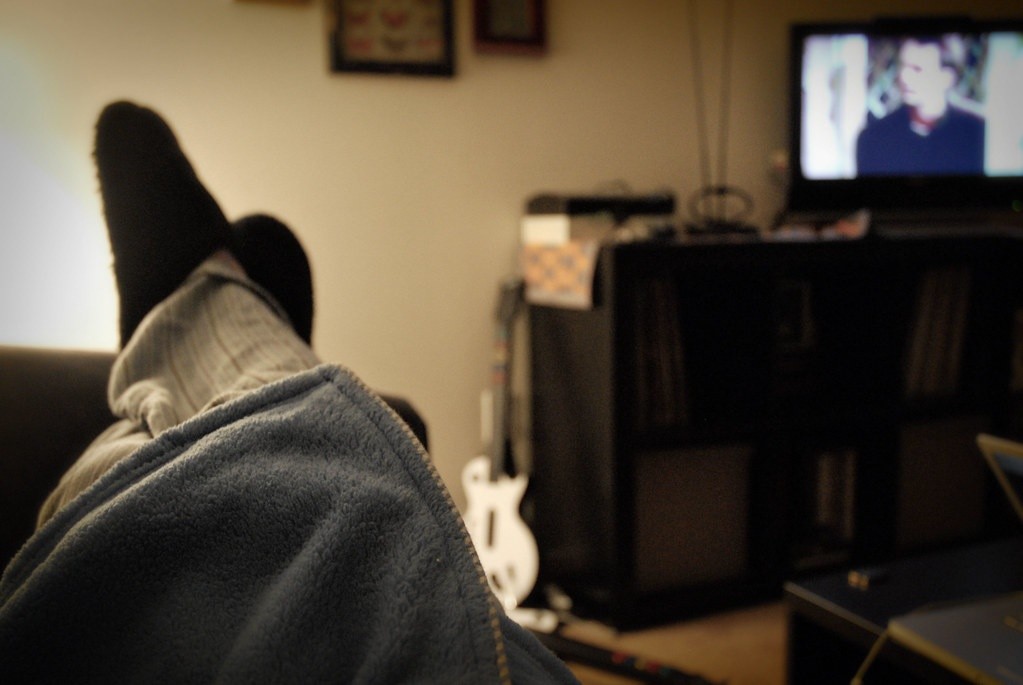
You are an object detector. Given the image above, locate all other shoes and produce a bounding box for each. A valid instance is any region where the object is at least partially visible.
[93,99,238,355]
[234,212,314,349]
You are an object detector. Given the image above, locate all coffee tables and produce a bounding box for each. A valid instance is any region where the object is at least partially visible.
[783,544,1023,685]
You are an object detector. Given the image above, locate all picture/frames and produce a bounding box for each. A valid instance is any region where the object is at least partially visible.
[326,0,455,76]
[474,0,547,52]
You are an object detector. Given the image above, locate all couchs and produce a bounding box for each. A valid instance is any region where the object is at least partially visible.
[0,348,433,584]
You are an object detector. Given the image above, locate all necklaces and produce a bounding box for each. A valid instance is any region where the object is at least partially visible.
[911,113,932,126]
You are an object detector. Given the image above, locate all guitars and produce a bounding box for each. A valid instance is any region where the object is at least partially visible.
[461,274,541,610]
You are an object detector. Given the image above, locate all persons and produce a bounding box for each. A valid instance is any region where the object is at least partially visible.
[0,102,583,685]
[857,34,984,173]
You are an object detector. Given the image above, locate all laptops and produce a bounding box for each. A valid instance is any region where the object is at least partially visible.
[885,434,1023,684]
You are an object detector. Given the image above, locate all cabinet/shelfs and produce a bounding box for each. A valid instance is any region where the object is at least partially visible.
[525,228,1023,626]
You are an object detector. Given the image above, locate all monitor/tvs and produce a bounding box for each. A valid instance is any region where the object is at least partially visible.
[786,13,1023,222]
